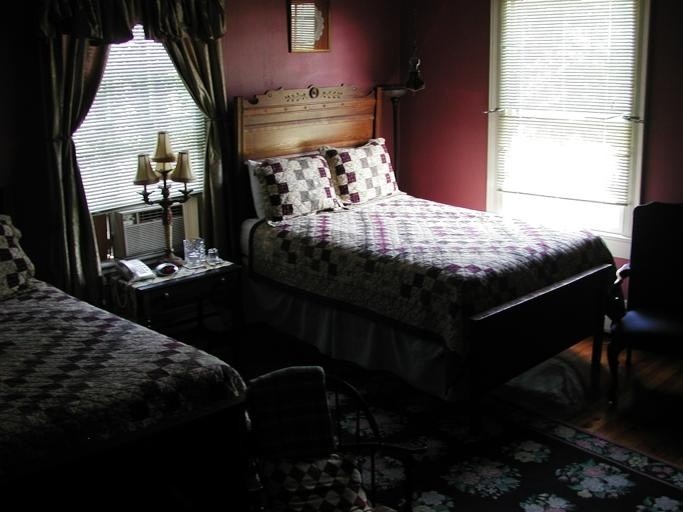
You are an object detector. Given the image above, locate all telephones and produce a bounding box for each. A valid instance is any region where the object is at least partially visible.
[117,259,155,283]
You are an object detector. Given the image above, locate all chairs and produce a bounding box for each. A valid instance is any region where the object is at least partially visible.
[607,201,683,408]
[244,364,412,512]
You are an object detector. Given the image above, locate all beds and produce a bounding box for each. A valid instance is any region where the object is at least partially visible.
[234,83,613,419]
[0,215,249,512]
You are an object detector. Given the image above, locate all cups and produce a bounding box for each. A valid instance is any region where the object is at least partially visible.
[183,238,207,270]
[208,249,219,266]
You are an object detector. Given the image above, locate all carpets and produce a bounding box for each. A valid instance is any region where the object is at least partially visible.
[327,380,683,512]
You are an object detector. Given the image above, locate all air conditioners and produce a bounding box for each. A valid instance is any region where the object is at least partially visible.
[109,203,186,261]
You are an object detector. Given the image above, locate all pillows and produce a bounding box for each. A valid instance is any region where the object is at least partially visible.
[248,138,407,228]
[0,215,35,298]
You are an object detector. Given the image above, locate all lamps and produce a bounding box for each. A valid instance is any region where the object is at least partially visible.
[134,131,196,270]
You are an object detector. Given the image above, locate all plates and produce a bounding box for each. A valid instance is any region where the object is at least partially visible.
[156,263,179,276]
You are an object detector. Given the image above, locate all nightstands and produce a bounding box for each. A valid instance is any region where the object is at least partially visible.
[104,246,242,347]
